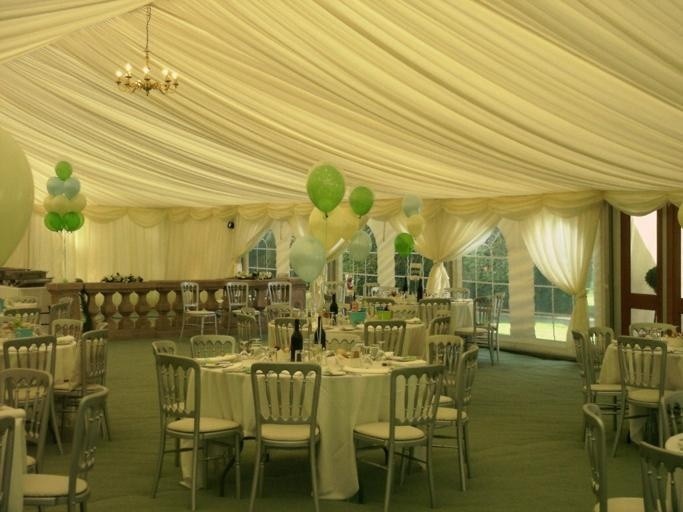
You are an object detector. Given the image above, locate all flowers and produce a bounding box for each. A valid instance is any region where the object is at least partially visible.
[235,269,274,279]
[101,272,144,283]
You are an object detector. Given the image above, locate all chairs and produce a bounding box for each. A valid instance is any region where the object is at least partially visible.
[5,307,41,326]
[364,322,406,358]
[588,327,613,382]
[49,328,110,445]
[422,317,451,360]
[350,365,444,512]
[454,297,497,369]
[609,336,683,458]
[152,340,193,467]
[572,329,636,440]
[19,384,109,512]
[473,291,505,363]
[400,346,479,491]
[151,352,243,511]
[268,281,293,319]
[582,403,671,511]
[248,362,321,511]
[362,295,397,315]
[630,323,678,339]
[226,280,263,339]
[188,335,235,359]
[51,321,83,340]
[179,281,218,338]
[275,316,312,347]
[4,335,65,456]
[425,335,464,409]
[0,367,54,512]
[49,301,72,322]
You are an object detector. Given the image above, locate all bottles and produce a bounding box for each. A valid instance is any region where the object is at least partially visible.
[314,316,326,351]
[330,295,338,325]
[290,319,303,362]
[402,277,409,298]
[416,279,423,302]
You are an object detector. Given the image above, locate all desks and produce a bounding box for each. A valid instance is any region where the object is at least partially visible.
[665,428,682,512]
[601,332,683,394]
[1,333,77,382]
[185,346,433,500]
[0,403,26,512]
[268,316,428,357]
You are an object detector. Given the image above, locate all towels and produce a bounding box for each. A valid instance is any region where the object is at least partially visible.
[222,363,245,373]
[365,368,391,375]
[56,335,73,345]
[222,353,241,361]
[385,351,395,359]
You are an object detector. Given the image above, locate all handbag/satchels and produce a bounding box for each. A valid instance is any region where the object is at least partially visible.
[404,317,420,324]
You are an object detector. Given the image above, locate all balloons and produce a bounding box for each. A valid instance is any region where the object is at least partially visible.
[350,187,373,218]
[288,238,327,284]
[307,164,345,219]
[402,194,423,218]
[53,195,69,216]
[43,195,54,212]
[69,194,87,212]
[340,209,359,241]
[65,178,80,199]
[394,232,414,257]
[0,131,33,270]
[58,223,65,231]
[77,212,85,230]
[47,177,64,195]
[350,230,372,262]
[406,215,425,239]
[309,205,345,249]
[64,213,80,231]
[45,213,60,232]
[55,161,72,180]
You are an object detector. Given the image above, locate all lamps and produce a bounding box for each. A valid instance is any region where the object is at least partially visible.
[117,0,181,97]
